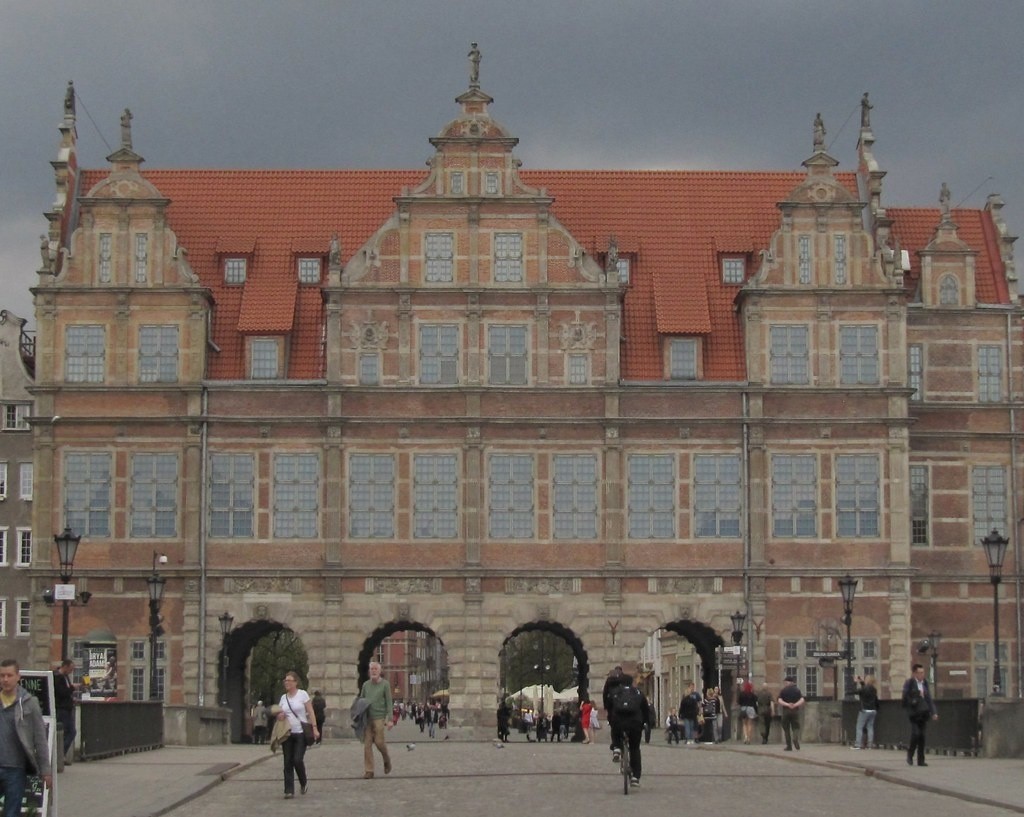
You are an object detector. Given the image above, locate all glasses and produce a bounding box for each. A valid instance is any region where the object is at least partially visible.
[282,679,295,683]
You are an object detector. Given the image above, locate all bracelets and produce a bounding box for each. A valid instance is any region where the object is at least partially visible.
[313,724,317,727]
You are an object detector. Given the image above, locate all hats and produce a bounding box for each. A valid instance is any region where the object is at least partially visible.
[783,675,793,682]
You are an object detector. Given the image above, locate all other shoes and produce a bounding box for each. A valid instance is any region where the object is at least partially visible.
[715,740,720,744]
[611,749,621,762]
[793,739,800,749]
[64,760,72,766]
[301,782,308,794]
[583,738,589,743]
[384,759,392,773]
[686,740,693,744]
[784,746,792,750]
[284,792,294,799]
[762,739,767,744]
[850,745,861,750]
[630,776,640,787]
[364,771,374,779]
[907,759,913,765]
[744,741,750,744]
[917,762,928,766]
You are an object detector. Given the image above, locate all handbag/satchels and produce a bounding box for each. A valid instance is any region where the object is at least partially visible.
[909,695,930,717]
[703,702,713,715]
[739,710,747,719]
[697,714,704,724]
[301,721,315,745]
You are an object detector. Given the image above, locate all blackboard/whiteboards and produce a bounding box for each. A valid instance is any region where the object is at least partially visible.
[0,716,54,817]
[0,670,56,817]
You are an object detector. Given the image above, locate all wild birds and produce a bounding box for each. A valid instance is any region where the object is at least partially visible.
[407,742,416,751]
[493,741,504,749]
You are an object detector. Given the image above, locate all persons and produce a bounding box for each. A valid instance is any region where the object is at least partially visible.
[275,671,320,799]
[776,675,806,751]
[756,681,776,743]
[120,109,134,150]
[64,79,75,115]
[851,673,878,751]
[251,700,267,744]
[312,690,327,746]
[602,667,656,789]
[861,92,874,128]
[0,658,52,817]
[677,681,728,744]
[52,659,82,764]
[525,699,601,744]
[468,43,483,84]
[360,660,394,779]
[813,112,826,144]
[392,699,450,738]
[665,707,680,744]
[939,181,951,213]
[739,678,758,744]
[903,663,939,766]
[497,700,514,742]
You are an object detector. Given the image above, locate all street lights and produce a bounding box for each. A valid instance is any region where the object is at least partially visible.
[145,550,168,700]
[838,571,859,686]
[732,611,747,678]
[219,613,234,706]
[920,631,943,699]
[534,663,550,718]
[54,522,83,662]
[980,528,1012,694]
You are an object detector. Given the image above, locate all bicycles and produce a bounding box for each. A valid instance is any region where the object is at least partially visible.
[607,723,648,796]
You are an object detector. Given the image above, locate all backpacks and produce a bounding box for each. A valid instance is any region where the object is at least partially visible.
[612,684,642,713]
